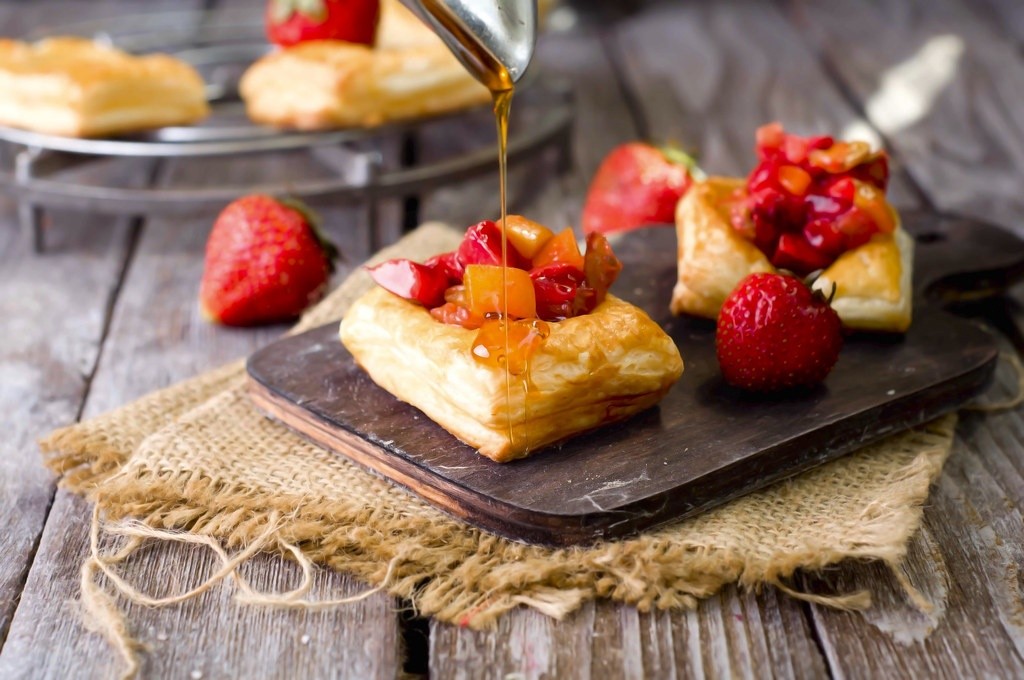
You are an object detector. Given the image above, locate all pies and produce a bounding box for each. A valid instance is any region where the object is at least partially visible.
[236,0,494,131]
[0,36,214,139]
[339,212,684,465]
[670,122,919,331]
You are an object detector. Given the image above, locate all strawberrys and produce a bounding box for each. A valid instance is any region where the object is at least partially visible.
[196,195,340,327]
[582,141,707,239]
[714,270,841,388]
[265,2,381,55]
